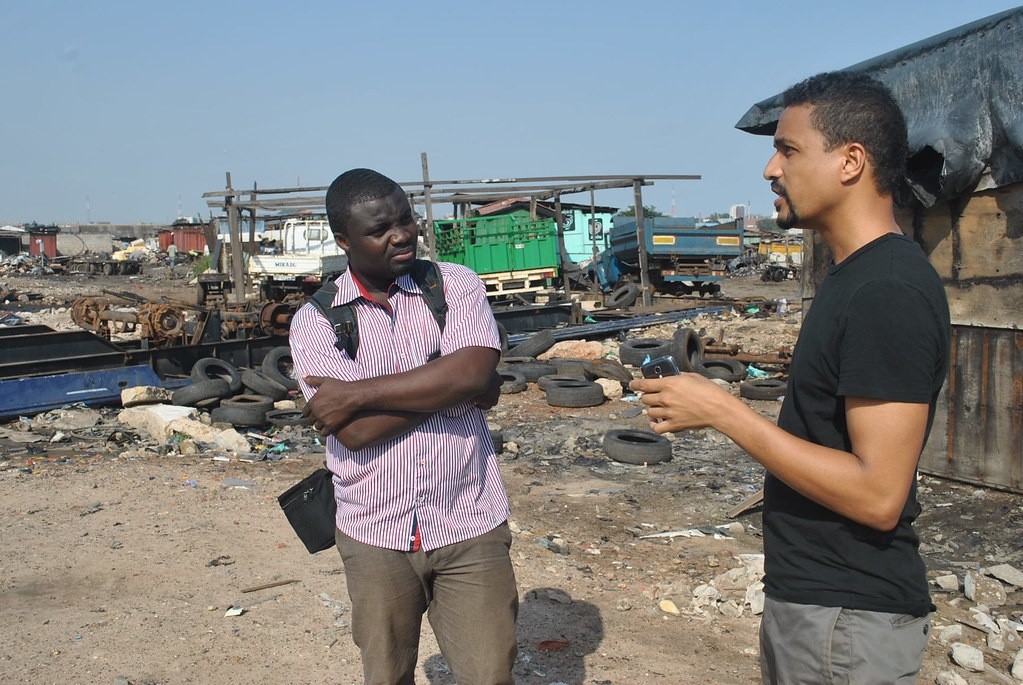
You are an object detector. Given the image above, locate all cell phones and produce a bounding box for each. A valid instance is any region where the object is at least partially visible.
[641,355,691,433]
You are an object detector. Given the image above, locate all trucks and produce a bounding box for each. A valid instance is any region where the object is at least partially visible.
[248,216,356,300]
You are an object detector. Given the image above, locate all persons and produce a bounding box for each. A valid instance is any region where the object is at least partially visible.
[289,168,519,685]
[167,241,178,272]
[630,68,952,684]
